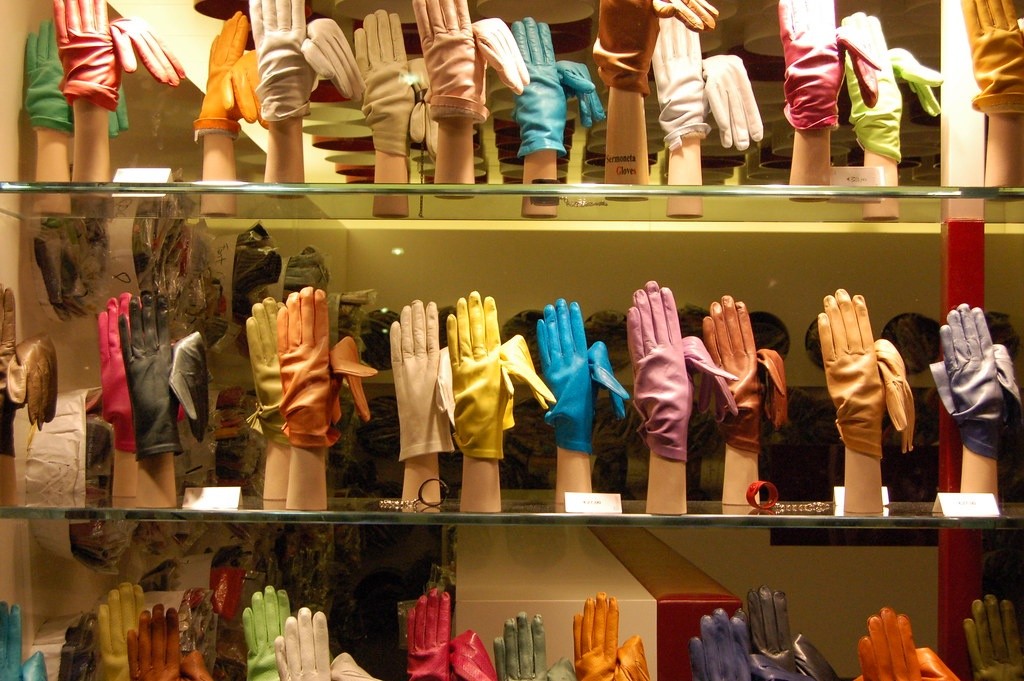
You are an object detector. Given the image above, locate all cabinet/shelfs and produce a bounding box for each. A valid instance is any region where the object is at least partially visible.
[0,0,1024,680]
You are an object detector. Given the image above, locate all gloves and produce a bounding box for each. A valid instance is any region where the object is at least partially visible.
[0,585,1024,680]
[20,1,1024,156]
[0,286,1023,462]
